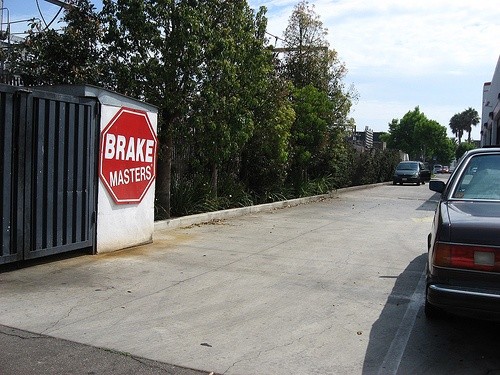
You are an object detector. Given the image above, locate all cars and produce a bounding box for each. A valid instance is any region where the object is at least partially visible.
[392,160,425,187]
[425,148,500,323]
[429,162,455,173]
